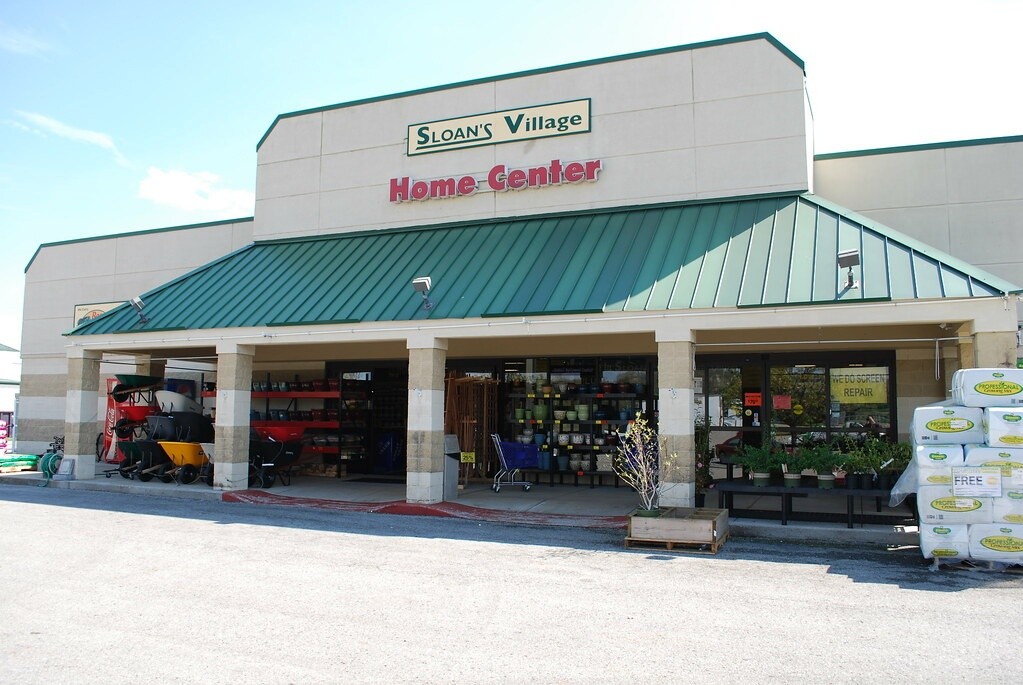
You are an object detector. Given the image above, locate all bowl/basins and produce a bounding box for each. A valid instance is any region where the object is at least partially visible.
[515,435,522,442]
[571,454,582,461]
[521,436,533,444]
[554,411,577,420]
[570,433,584,444]
[583,454,591,461]
[534,434,546,445]
[580,461,591,471]
[559,384,568,392]
[543,387,553,393]
[569,461,581,470]
[523,429,533,436]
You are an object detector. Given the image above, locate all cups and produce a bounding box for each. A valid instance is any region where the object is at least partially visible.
[515,408,525,419]
[525,411,533,419]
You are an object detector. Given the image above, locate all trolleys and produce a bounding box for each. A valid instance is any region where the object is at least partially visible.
[491,433,540,492]
[109,375,316,488]
